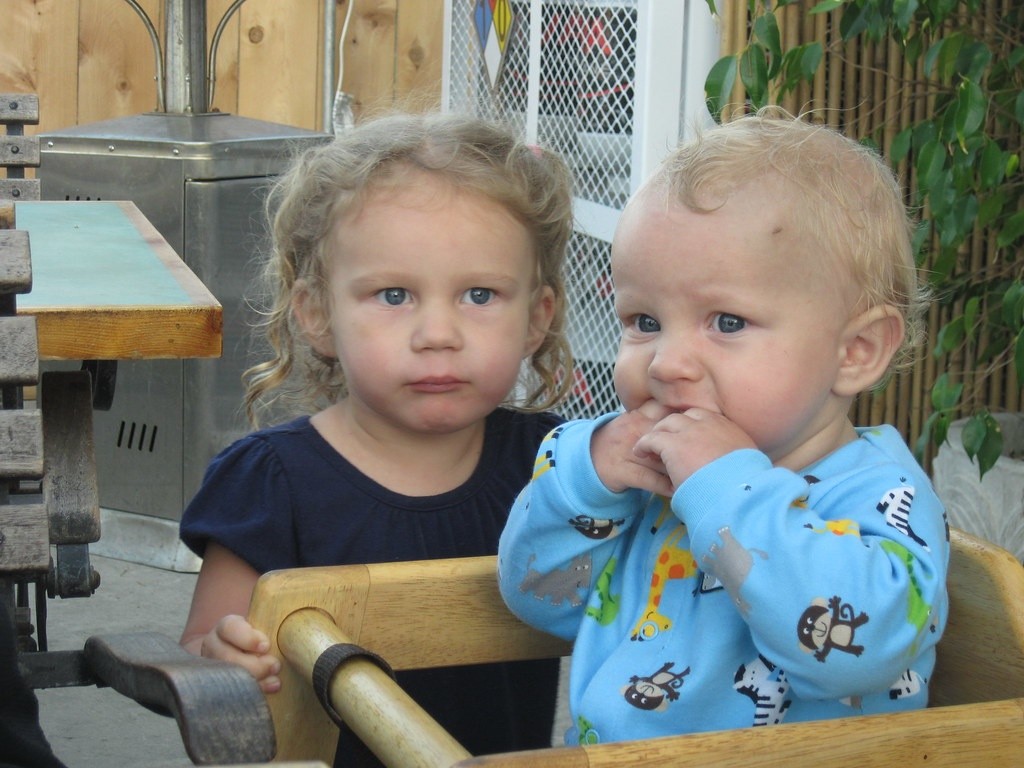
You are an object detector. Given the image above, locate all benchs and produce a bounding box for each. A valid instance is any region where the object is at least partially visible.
[0,94,277,768]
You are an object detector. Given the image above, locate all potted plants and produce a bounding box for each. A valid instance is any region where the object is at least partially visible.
[705,0,1024,566]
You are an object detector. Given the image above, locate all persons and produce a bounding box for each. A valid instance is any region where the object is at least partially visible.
[497,119,949,747]
[178,113,572,768]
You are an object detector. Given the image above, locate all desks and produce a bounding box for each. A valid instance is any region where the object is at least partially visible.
[15,200,223,360]
